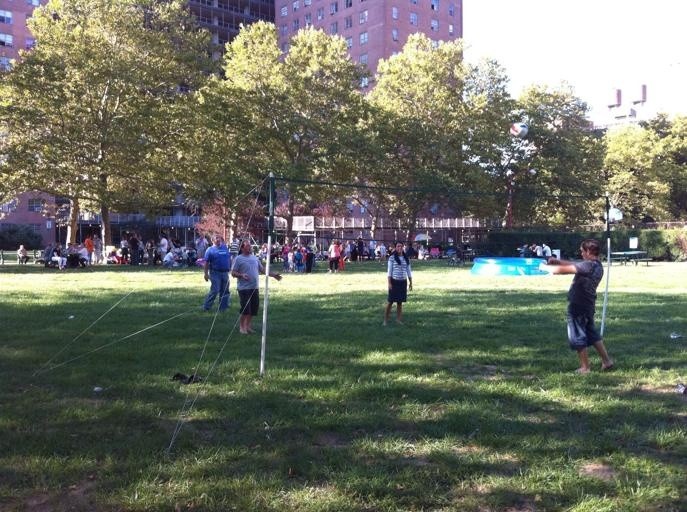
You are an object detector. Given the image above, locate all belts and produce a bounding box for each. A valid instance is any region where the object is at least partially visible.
[210,267,230,272]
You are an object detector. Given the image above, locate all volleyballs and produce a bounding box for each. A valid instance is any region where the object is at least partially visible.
[510,122,528,138]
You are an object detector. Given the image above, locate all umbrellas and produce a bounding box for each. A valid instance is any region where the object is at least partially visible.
[413,234,432,242]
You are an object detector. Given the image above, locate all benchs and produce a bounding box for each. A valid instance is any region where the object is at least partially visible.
[607,250,654,266]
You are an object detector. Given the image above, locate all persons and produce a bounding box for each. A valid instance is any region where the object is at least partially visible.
[201,233,233,312]
[382,241,412,327]
[516,242,552,260]
[229,239,282,335]
[17,244,29,264]
[538,238,613,373]
[36,231,419,274]
